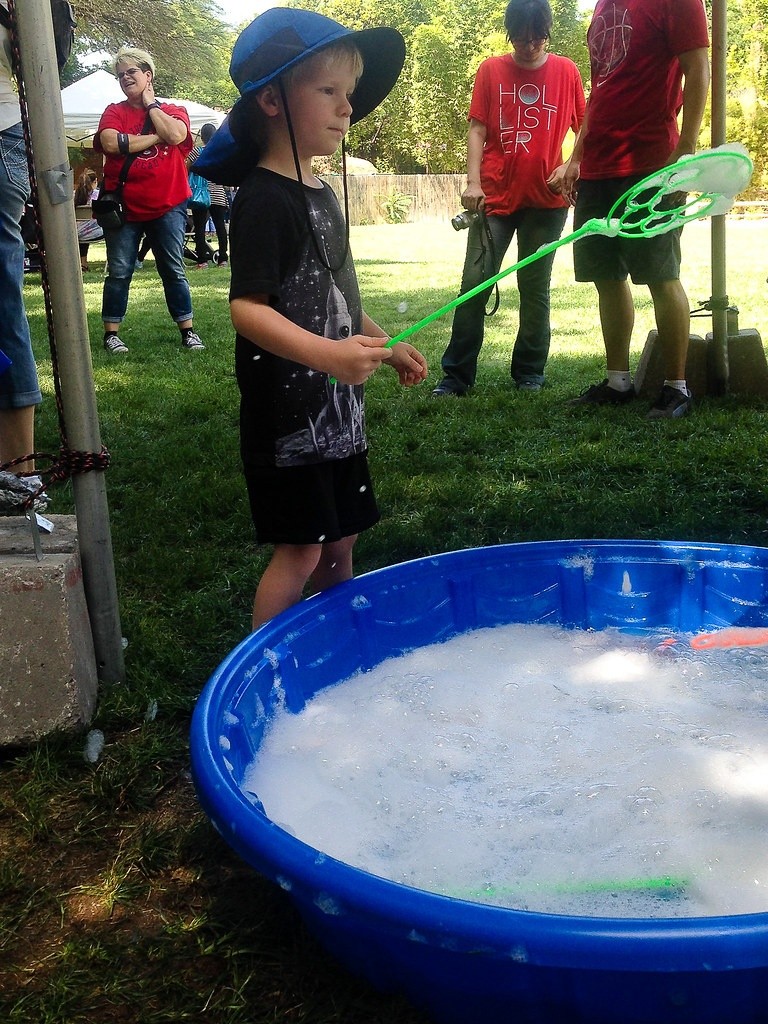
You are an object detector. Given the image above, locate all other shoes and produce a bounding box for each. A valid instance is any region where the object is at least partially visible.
[515,382,543,396]
[430,383,457,399]
[196,263,210,272]
[216,261,228,270]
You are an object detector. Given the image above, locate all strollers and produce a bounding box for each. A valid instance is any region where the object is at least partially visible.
[183,211,221,267]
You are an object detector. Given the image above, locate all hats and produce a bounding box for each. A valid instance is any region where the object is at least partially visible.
[182,6,411,193]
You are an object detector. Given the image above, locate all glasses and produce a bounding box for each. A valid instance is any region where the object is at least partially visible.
[502,33,551,50]
[114,68,149,80]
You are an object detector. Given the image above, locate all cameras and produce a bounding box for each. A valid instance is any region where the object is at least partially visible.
[451,209,484,231]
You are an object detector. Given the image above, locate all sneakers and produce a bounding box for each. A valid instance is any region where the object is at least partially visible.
[562,377,636,409]
[644,385,694,423]
[102,329,129,354]
[181,329,205,351]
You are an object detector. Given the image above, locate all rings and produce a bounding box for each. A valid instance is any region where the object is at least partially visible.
[148,88,152,92]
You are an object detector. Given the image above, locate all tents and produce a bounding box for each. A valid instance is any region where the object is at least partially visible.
[58,68,227,149]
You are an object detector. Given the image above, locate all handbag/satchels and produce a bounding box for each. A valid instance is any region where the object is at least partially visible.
[182,168,212,209]
[91,184,127,231]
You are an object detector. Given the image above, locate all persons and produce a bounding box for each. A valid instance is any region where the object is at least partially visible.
[561,0,711,421]
[92,46,207,355]
[187,124,239,268]
[188,7,428,631]
[430,0,587,396]
[0,0,47,511]
[74,169,105,272]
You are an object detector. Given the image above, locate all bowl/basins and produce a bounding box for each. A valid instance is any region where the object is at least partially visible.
[189,539,768,1024]
